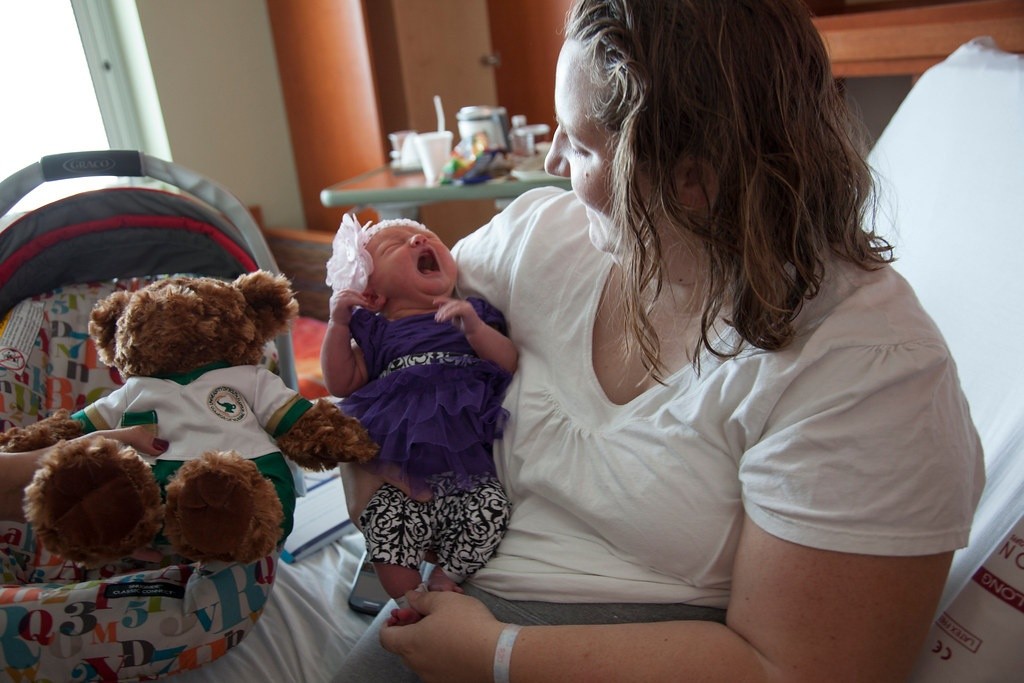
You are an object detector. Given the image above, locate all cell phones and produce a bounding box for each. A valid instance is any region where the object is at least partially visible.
[348,551,391,615]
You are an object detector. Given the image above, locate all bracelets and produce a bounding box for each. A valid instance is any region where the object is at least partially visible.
[494,625,523,683]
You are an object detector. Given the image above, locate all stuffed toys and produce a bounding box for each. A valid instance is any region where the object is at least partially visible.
[0,269,381,564]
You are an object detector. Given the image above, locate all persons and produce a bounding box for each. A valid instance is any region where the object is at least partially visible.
[321,213,518,627]
[161,0,988,683]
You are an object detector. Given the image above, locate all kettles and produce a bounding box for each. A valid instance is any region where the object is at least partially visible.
[456,106,509,152]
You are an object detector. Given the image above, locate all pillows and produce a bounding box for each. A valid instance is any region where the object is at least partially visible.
[861,35,1021,629]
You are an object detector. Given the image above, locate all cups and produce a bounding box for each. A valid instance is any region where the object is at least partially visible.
[415,132,454,181]
[388,130,416,169]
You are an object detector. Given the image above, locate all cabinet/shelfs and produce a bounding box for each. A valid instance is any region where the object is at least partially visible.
[264,0,579,234]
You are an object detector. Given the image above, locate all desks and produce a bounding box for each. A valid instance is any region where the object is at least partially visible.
[319,141,573,222]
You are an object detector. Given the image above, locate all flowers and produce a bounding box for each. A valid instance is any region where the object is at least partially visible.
[323,212,375,293]
